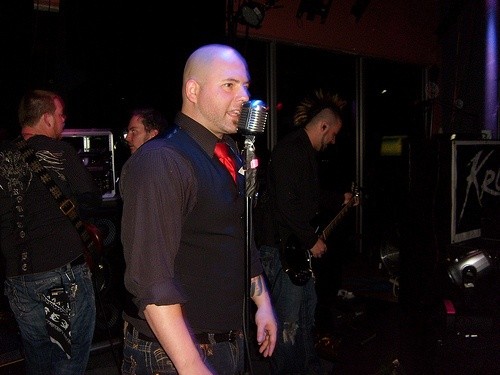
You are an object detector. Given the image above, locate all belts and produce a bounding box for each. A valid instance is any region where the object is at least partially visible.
[127,322,239,344]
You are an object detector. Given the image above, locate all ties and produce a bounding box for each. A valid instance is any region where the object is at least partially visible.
[214,141,238,186]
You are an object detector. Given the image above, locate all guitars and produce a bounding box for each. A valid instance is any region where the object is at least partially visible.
[279,182,361,287]
[81,222,103,270]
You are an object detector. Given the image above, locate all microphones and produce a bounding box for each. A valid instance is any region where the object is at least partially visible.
[238,100,268,178]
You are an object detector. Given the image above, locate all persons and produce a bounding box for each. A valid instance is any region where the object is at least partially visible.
[118,44,278,375]
[127,109,170,153]
[252,89,360,375]
[0,90,102,375]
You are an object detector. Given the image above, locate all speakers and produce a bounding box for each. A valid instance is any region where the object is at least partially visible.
[81,205,139,344]
